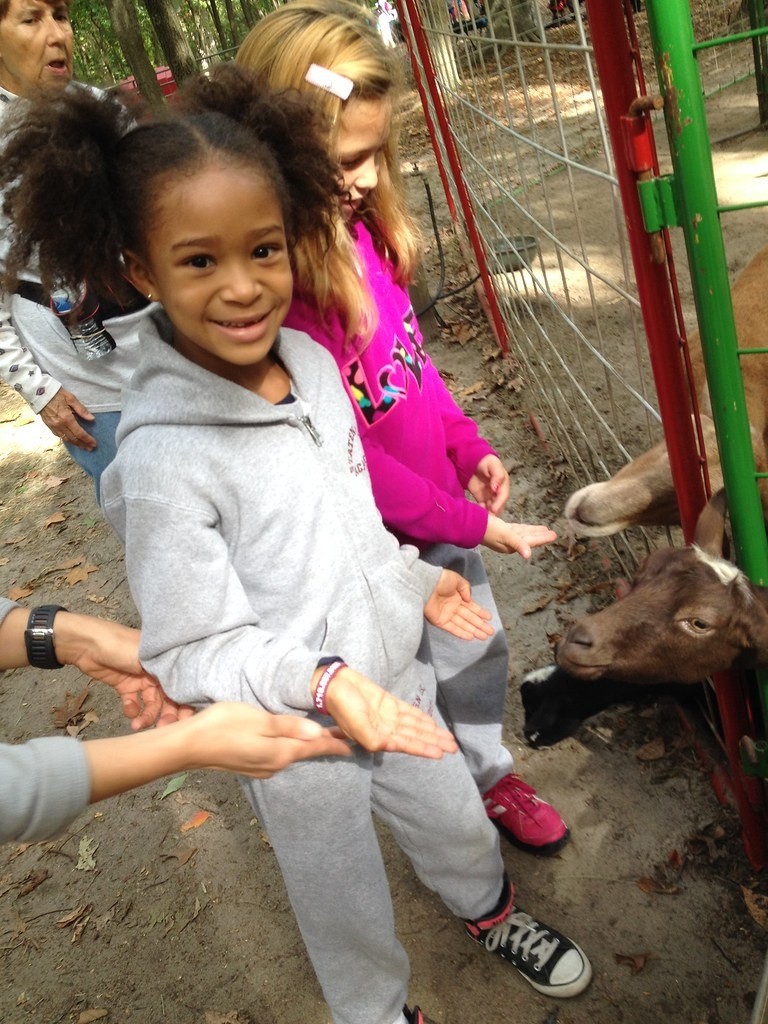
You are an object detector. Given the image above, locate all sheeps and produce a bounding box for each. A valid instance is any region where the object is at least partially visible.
[553,249,768,689]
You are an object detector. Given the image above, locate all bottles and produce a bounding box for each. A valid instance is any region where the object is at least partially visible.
[45,257,115,363]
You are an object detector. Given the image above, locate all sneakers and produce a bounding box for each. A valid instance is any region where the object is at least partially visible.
[482,773,570,856]
[403,1003,435,1024]
[462,871,592,998]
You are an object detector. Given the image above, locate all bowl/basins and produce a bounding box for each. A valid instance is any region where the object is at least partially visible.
[483,237,541,274]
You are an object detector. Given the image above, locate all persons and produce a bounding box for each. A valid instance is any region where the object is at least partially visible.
[225,8,576,864]
[0,63,593,1022]
[0,595,354,845]
[0,0,150,515]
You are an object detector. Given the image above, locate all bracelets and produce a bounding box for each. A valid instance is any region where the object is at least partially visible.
[314,659,349,714]
[23,604,68,669]
